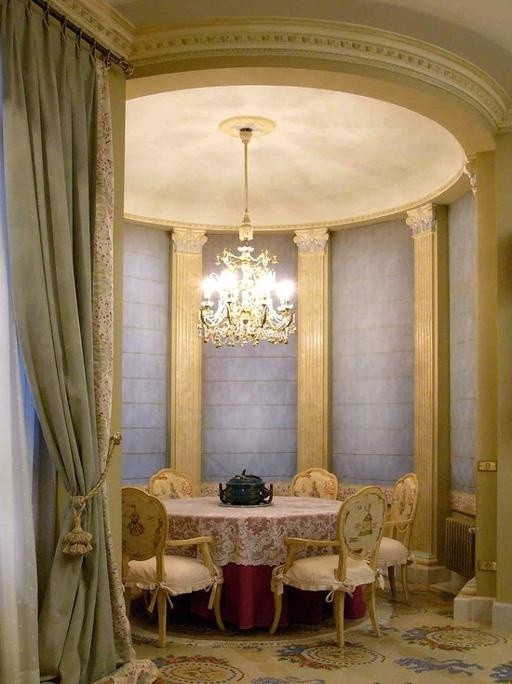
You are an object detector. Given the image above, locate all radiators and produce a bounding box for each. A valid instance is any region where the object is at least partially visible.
[443,516,475,579]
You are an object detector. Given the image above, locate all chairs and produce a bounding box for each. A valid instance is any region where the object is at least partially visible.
[122,486,226,649]
[269,486,388,648]
[377,472,420,608]
[290,467,338,500]
[148,468,193,499]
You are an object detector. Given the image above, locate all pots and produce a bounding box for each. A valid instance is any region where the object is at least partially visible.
[218,468,274,507]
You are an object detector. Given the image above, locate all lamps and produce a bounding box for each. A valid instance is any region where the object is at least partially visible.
[197,116,297,349]
